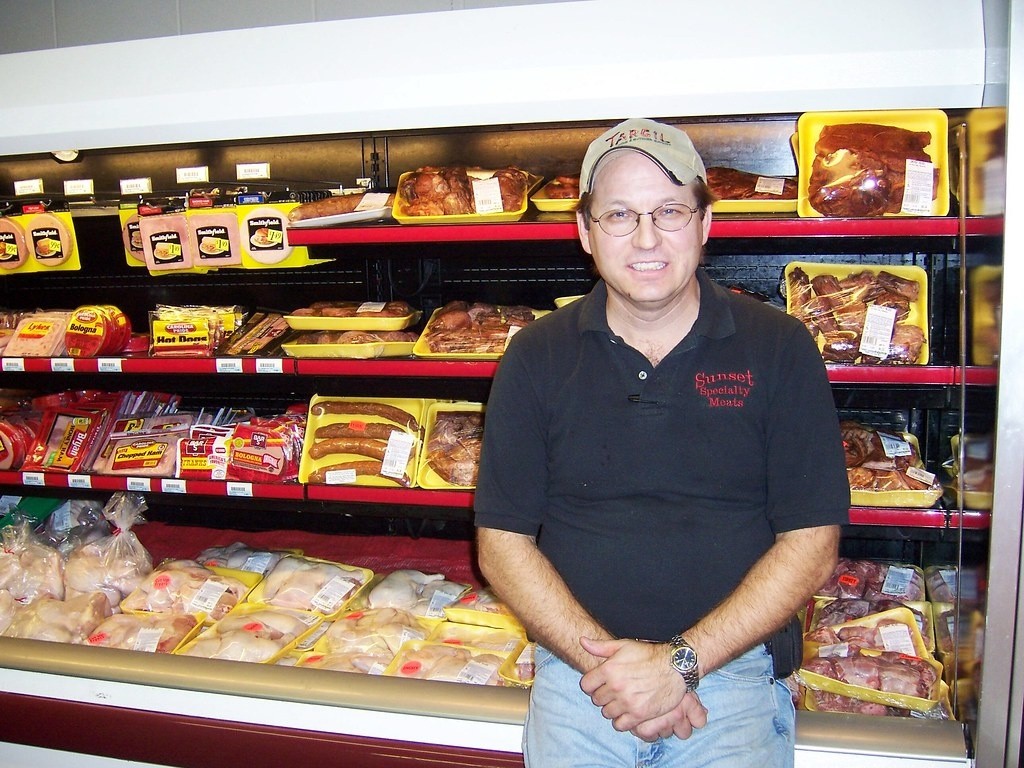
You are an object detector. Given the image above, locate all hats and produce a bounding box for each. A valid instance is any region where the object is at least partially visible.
[578,118,708,200]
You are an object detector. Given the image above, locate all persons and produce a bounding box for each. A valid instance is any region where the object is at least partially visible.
[472,117,852,766]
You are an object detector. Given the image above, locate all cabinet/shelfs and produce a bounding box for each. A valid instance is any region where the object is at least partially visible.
[0,109,1024,768]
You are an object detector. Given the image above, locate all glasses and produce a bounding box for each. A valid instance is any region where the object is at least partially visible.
[589,202,701,237]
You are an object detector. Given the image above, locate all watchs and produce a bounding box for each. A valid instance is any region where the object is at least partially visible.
[667,635,702,694]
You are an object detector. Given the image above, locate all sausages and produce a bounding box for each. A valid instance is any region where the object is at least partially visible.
[310,400,417,487]
